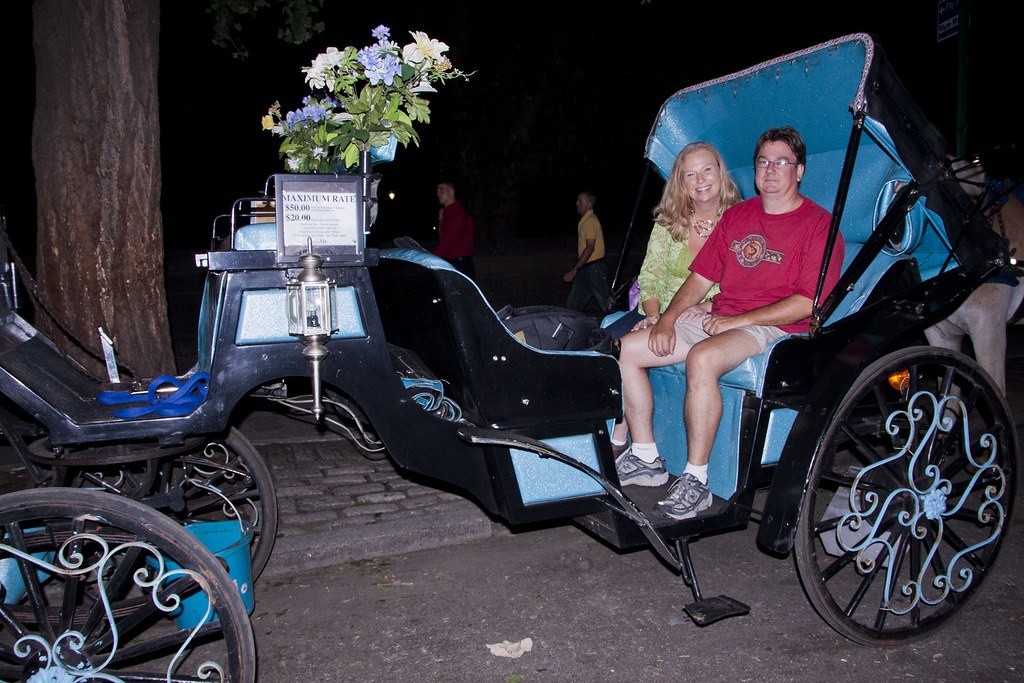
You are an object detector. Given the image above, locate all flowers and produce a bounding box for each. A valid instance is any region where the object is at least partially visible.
[261,25,478,175]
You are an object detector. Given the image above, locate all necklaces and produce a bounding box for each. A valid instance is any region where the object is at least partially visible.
[691,216,715,238]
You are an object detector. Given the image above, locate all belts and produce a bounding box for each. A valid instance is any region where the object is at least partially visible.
[582,258,604,268]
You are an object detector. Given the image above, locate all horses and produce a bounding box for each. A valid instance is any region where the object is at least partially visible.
[916,154,1024,444]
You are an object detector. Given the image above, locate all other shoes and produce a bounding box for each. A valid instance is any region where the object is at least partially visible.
[611,442,630,464]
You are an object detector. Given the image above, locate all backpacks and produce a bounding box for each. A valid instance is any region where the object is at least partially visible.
[498,304,613,355]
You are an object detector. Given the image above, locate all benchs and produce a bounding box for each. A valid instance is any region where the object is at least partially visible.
[368,247,623,505]
[599,238,871,501]
[209,133,398,251]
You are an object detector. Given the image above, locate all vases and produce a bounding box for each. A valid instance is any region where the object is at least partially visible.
[360,149,372,234]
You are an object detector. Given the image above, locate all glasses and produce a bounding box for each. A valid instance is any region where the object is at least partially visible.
[757,156,799,168]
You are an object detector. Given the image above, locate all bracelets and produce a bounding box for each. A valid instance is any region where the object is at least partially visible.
[573,265,579,271]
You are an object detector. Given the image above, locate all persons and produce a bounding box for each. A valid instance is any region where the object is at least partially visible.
[615,125,844,520]
[603,141,744,459]
[559,191,610,314]
[428,178,474,277]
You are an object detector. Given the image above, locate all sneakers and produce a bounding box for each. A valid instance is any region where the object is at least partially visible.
[616,449,670,487]
[652,472,713,520]
